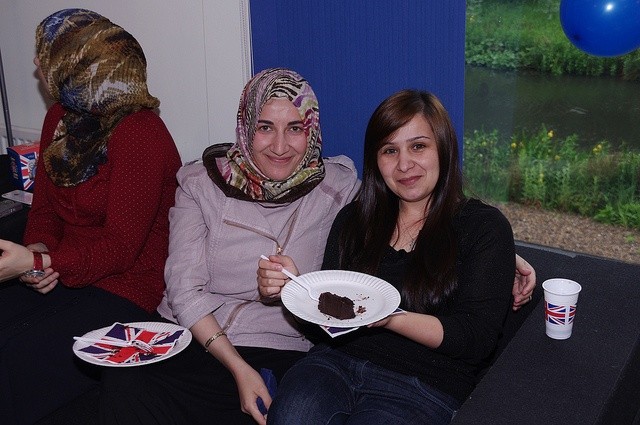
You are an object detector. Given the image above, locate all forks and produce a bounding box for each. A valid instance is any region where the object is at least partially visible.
[259,254,321,302]
[73,336,153,353]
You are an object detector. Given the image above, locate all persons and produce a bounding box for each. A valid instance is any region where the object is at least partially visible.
[255,89,516,425]
[97,65,538,425]
[0,7,182,425]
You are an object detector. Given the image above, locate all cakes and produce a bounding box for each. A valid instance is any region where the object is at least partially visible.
[318,292,356,319]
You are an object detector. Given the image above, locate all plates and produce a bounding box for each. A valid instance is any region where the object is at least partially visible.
[281,270,403,327]
[72,322,192,366]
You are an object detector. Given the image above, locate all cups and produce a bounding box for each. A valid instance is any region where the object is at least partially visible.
[542,278,583,340]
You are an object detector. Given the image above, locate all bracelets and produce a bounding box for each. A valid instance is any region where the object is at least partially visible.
[201,331,228,353]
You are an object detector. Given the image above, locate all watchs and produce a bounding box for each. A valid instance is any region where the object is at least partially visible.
[25,251,45,278]
